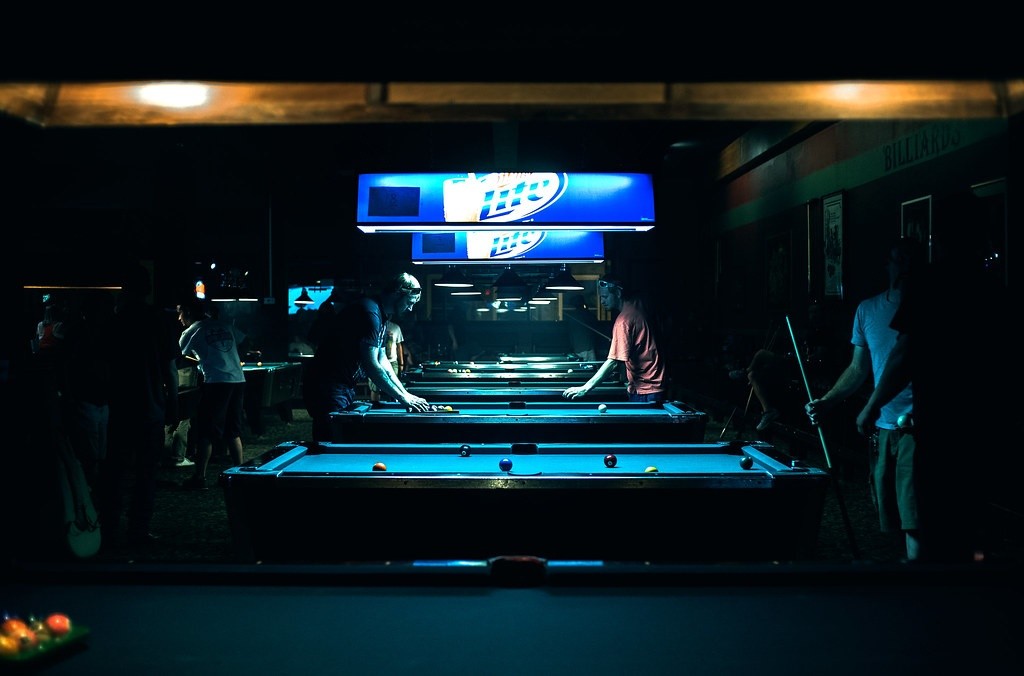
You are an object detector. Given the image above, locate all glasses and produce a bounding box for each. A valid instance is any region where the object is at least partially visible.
[403,287,423,295]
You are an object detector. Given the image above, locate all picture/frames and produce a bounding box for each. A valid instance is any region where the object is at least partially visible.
[969,177,1009,288]
[819,188,848,303]
[897,194,934,276]
[763,229,793,309]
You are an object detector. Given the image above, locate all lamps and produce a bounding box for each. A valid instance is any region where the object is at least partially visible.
[209,262,259,301]
[294,286,314,304]
[434,264,585,313]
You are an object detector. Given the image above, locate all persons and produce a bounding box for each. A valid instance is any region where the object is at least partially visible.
[36,286,246,543]
[301,270,430,440]
[446,311,481,362]
[568,295,599,359]
[562,274,666,400]
[729,189,1009,566]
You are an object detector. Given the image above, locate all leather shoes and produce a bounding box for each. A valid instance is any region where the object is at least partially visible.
[126,526,162,544]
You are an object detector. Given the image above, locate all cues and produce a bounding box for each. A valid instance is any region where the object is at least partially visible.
[719,319,782,440]
[784,314,861,558]
[562,311,611,341]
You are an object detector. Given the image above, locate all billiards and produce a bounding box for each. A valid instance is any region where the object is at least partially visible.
[0,610,72,656]
[499,458,513,471]
[604,454,617,467]
[897,414,909,428]
[740,456,753,470]
[598,404,607,412]
[257,362,262,366]
[447,368,471,373]
[644,466,659,473]
[373,462,387,471]
[407,404,453,413]
[460,445,471,456]
[240,362,246,366]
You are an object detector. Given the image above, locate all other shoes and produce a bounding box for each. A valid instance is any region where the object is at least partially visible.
[173,457,196,466]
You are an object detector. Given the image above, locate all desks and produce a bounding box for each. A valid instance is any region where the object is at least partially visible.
[0,348,1024,676]
